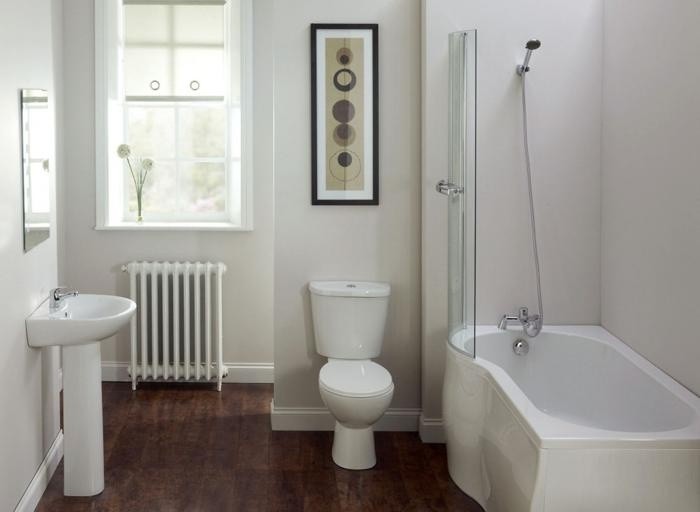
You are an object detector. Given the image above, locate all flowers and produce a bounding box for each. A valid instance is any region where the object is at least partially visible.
[117,144,157,220]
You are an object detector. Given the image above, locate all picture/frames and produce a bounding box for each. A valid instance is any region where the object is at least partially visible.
[309,22,382,207]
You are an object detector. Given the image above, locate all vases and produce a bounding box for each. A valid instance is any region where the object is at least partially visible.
[133,183,146,224]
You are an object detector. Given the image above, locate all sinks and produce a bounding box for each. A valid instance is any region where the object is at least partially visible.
[26,292,137,347]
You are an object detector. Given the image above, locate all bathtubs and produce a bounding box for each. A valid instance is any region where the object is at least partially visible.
[441,322,700,512]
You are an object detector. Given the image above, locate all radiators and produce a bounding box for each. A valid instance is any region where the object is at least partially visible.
[121,259,230,393]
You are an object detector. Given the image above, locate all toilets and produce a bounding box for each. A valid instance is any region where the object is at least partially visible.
[307,280,395,472]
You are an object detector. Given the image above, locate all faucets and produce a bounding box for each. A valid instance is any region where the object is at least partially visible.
[49,286,79,310]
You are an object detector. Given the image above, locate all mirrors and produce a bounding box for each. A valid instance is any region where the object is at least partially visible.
[20,88,58,253]
